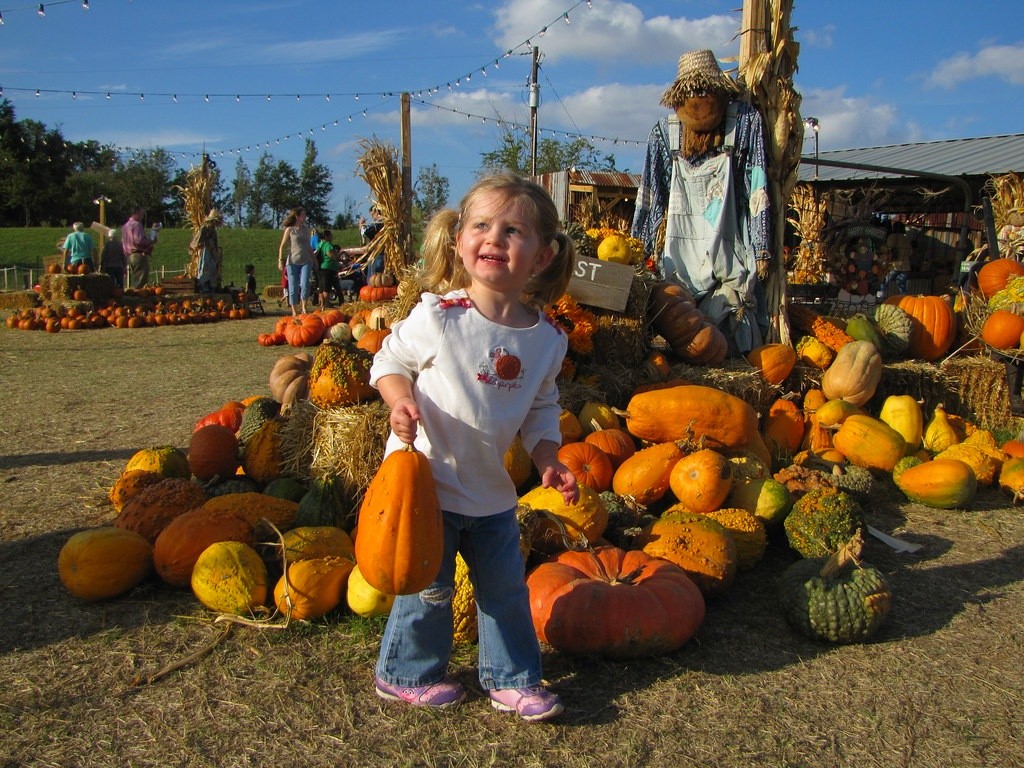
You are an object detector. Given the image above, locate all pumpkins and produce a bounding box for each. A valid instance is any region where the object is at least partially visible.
[6,263,250,333]
[56,222,1024,660]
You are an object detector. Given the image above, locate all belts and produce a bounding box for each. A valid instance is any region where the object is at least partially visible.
[132,250,144,253]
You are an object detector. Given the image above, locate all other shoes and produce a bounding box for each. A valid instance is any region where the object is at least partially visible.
[277,299,282,306]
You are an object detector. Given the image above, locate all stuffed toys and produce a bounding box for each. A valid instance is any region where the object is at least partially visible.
[674,79,729,129]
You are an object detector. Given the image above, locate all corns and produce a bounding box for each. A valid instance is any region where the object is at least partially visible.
[772,108,789,164]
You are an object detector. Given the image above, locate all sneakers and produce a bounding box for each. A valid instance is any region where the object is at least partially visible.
[489,683,564,719]
[376,673,467,707]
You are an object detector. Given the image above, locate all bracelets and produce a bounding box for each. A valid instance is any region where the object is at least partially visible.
[278,258,284,261]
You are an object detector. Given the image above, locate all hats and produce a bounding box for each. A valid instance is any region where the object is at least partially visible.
[658,50,741,108]
[205,210,221,221]
[108,229,117,237]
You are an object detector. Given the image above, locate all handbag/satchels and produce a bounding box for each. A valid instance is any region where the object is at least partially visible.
[885,234,899,261]
[313,250,323,267]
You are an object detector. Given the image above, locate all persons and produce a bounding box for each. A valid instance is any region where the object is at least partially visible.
[191,209,225,294]
[310,228,350,307]
[359,205,386,285]
[62,222,100,274]
[278,207,319,317]
[121,205,161,289]
[245,264,256,295]
[368,173,580,722]
[348,256,368,302]
[870,216,913,280]
[100,229,127,290]
